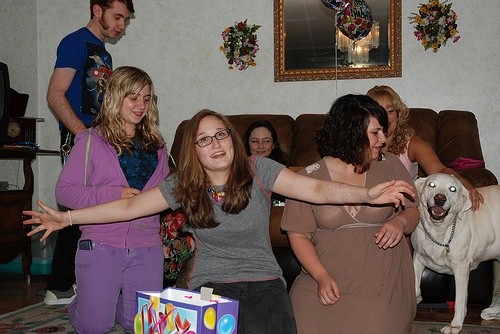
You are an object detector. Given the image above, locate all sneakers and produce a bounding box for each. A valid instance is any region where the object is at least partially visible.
[44,283,78,305]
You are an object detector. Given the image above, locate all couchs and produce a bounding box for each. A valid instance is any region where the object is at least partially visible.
[162,109,498,307]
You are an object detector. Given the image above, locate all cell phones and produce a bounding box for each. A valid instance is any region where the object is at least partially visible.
[79,239,92,250]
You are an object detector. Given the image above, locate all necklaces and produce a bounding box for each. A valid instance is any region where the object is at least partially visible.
[206,178,226,204]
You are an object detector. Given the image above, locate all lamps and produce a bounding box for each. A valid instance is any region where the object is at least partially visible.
[335,10,380,66]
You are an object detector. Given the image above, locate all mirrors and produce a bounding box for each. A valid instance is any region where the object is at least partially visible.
[273,0,403,83]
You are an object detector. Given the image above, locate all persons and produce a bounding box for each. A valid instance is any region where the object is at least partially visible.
[243,120,289,202]
[43,0,135,305]
[367,85,485,211]
[280,94,422,334]
[23,109,416,334]
[55,66,170,334]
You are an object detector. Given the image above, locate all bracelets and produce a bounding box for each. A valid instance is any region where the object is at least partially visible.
[67,210,73,226]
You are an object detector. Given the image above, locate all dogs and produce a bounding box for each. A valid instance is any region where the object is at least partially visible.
[413,172,500,334]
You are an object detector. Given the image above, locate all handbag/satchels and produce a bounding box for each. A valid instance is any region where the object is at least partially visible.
[132,286,240,334]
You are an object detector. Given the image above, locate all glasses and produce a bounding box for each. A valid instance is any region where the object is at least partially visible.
[194,129,231,147]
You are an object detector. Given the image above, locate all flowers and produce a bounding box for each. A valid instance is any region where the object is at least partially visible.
[337,0,374,40]
[159,211,195,281]
[219,19,263,70]
[408,0,461,54]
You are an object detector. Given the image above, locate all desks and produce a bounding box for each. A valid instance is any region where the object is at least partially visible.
[0,148,61,287]
